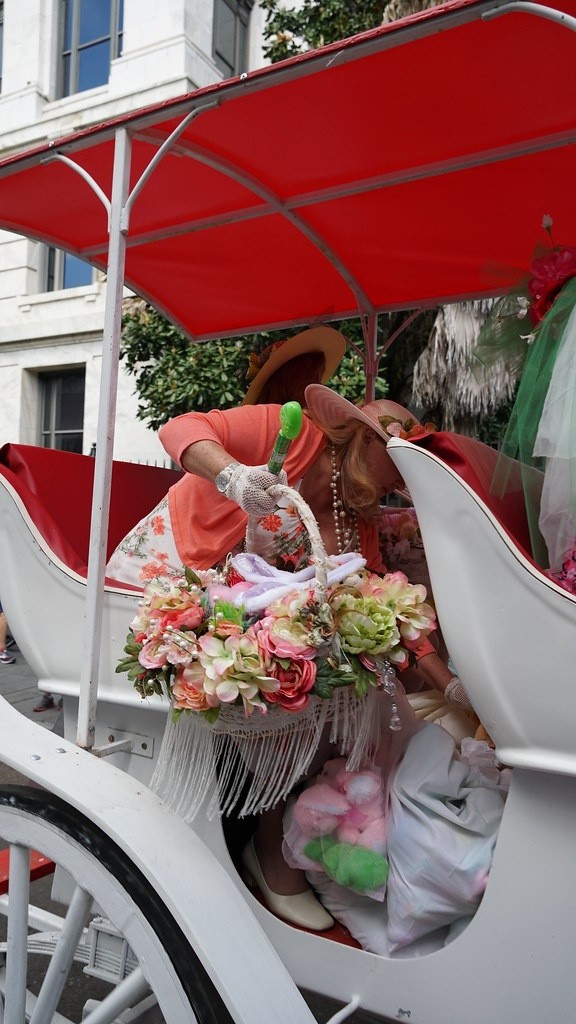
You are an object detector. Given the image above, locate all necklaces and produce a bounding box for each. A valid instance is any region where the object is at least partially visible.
[331,439,404,731]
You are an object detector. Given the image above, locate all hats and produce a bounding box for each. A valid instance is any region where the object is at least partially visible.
[304,383,422,444]
[241,326,346,406]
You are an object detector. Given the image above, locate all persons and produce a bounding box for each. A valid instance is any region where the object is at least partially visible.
[0,599,16,665]
[104,383,480,933]
[32,691,64,712]
[240,326,348,411]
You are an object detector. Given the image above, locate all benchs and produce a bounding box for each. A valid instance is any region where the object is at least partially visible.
[0,442,188,595]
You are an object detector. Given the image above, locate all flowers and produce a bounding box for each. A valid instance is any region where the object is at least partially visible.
[115,567,441,720]
[375,507,422,560]
[541,549,576,593]
[374,411,435,440]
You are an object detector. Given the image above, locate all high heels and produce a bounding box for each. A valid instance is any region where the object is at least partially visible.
[242,832,335,931]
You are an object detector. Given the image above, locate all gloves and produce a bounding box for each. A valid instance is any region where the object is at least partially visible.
[225,464,289,516]
[443,677,480,724]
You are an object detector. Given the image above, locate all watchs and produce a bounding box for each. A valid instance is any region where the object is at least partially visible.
[213,461,242,495]
